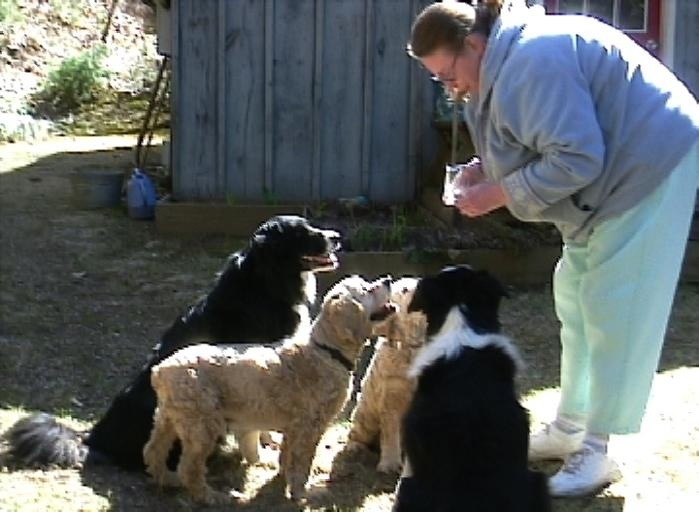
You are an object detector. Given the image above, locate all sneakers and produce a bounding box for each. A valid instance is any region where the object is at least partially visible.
[545,445,610,497]
[528,423,586,461]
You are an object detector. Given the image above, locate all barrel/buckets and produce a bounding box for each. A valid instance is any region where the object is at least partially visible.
[124,168,156,219]
[67,170,123,209]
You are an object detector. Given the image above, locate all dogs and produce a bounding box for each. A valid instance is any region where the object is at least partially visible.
[338,276,428,477]
[390,263,550,512]
[0,215,341,472]
[143,274,399,509]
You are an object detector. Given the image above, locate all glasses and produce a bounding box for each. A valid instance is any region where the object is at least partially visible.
[430,55,458,83]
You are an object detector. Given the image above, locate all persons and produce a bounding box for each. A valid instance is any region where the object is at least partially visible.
[405,0,699,497]
